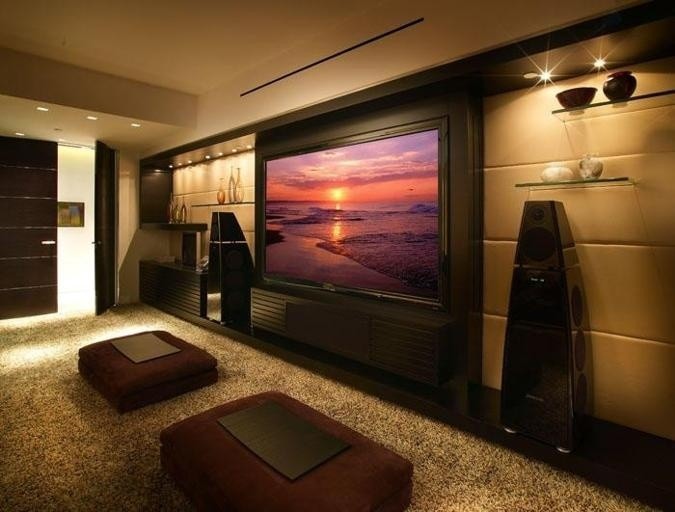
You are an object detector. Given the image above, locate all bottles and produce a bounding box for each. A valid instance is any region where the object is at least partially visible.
[234,168,246,203]
[602,70,638,109]
[165,191,187,224]
[216,178,226,205]
[578,152,605,181]
[228,166,237,205]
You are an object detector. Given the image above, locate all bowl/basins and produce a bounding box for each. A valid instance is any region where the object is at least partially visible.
[556,86,598,116]
[540,167,574,183]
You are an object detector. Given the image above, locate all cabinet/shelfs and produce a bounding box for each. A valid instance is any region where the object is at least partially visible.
[248,285,460,390]
[138,261,207,318]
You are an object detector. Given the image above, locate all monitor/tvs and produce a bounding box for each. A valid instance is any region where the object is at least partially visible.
[255,115,449,315]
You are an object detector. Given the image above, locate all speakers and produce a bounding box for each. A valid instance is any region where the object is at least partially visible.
[499,200,594,455]
[204,211,255,326]
